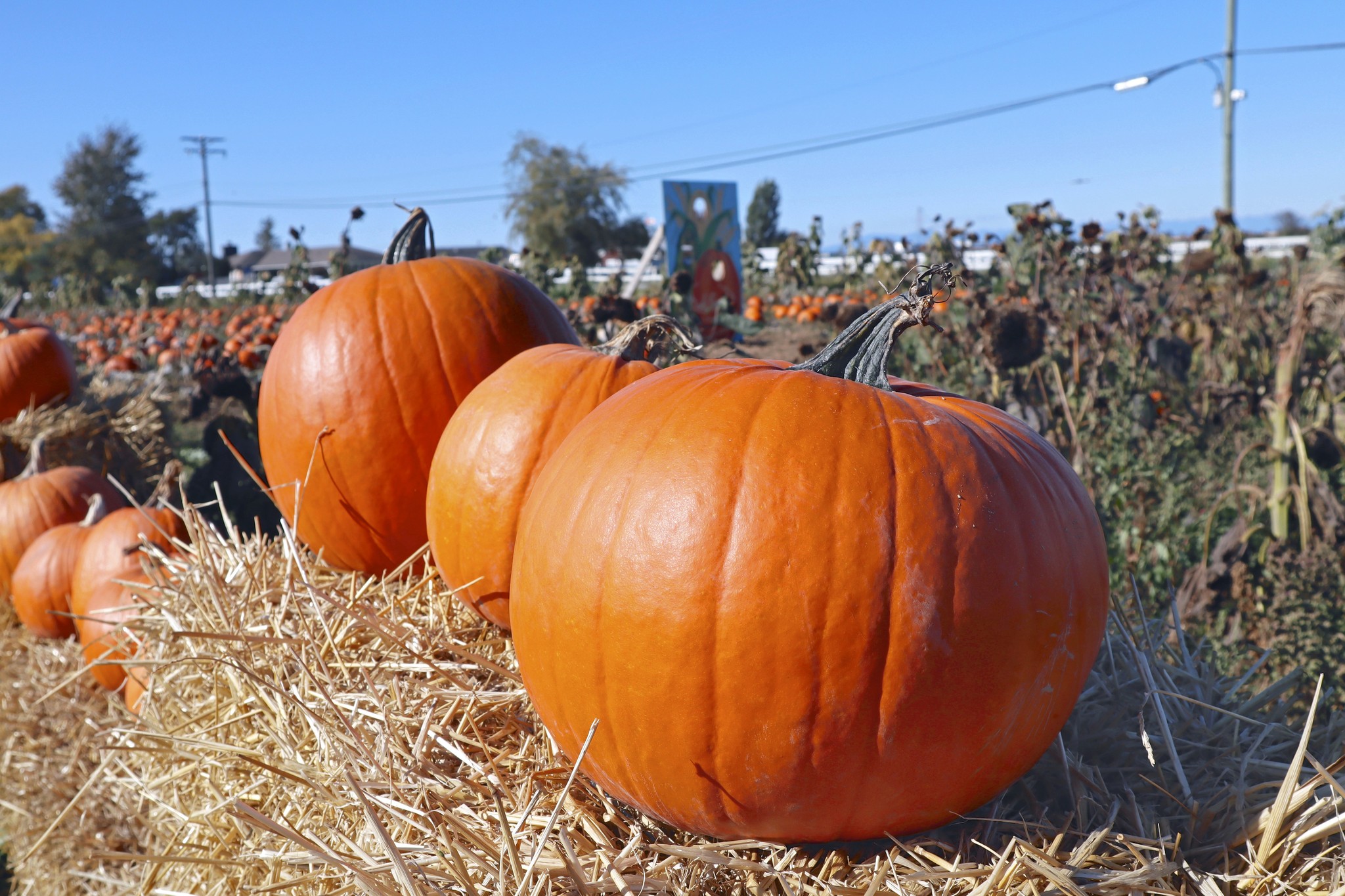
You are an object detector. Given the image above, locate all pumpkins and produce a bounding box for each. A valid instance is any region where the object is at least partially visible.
[1,208,1114,848]
